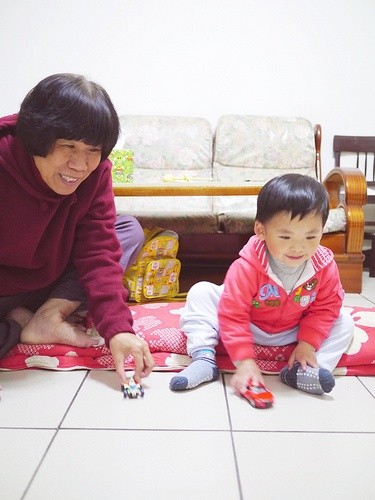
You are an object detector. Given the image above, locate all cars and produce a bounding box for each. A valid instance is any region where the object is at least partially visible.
[239,379,274,409]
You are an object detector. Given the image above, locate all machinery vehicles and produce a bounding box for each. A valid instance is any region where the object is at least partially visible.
[121,377,144,398]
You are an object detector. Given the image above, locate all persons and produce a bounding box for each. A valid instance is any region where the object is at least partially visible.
[168,173,356,392]
[0,72,154,388]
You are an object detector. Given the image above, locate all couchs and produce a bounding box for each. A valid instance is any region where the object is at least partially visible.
[107,111,369,294]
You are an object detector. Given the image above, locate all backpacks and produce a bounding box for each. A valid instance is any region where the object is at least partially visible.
[125,226,188,302]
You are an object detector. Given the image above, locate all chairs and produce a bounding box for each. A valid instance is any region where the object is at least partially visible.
[333,136,375,277]
